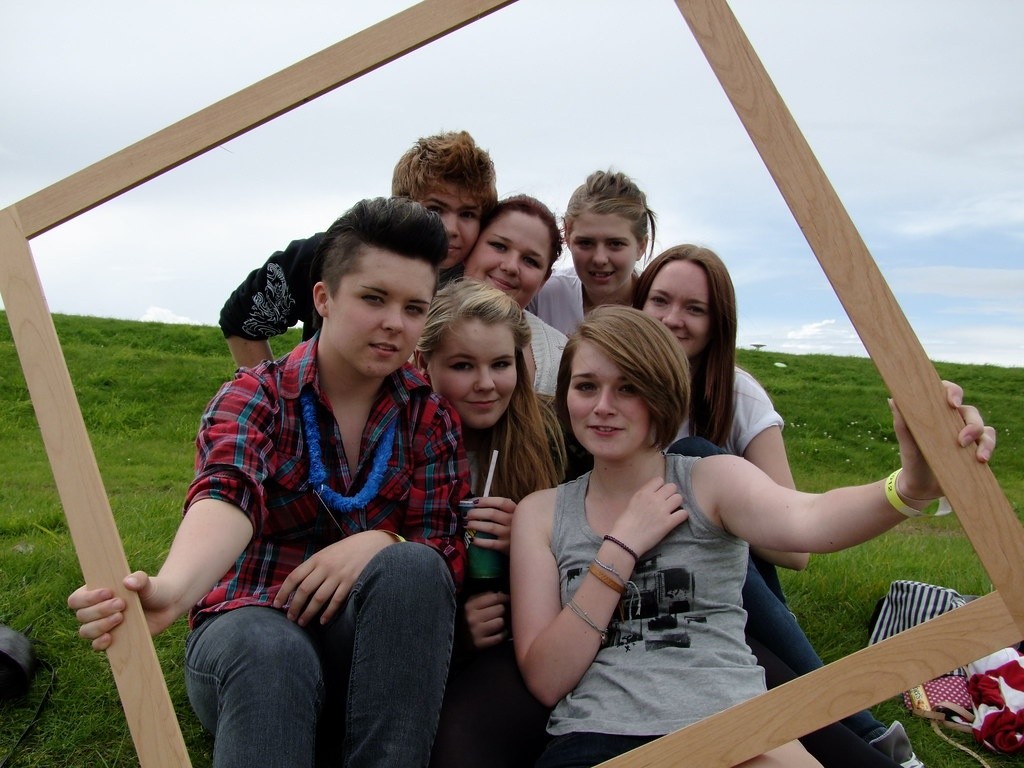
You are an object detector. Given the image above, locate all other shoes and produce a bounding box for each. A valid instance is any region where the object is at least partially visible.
[867,720,926,768]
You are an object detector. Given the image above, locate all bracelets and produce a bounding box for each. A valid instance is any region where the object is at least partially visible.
[592,556,642,624]
[566,597,608,644]
[603,534,639,563]
[885,467,954,518]
[896,471,940,503]
[589,565,630,624]
[375,528,406,544]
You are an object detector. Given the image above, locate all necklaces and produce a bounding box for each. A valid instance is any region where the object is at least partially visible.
[300,388,396,511]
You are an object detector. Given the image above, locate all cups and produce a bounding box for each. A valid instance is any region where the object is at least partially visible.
[459,498,502,578]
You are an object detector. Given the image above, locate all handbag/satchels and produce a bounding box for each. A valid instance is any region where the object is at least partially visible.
[0,625,35,697]
[866,579,1021,722]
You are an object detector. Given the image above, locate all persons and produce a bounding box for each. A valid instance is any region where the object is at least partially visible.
[407,276,595,767]
[219,130,499,376]
[633,243,926,768]
[517,172,655,341]
[509,302,995,768]
[461,194,572,399]
[69,194,470,767]
